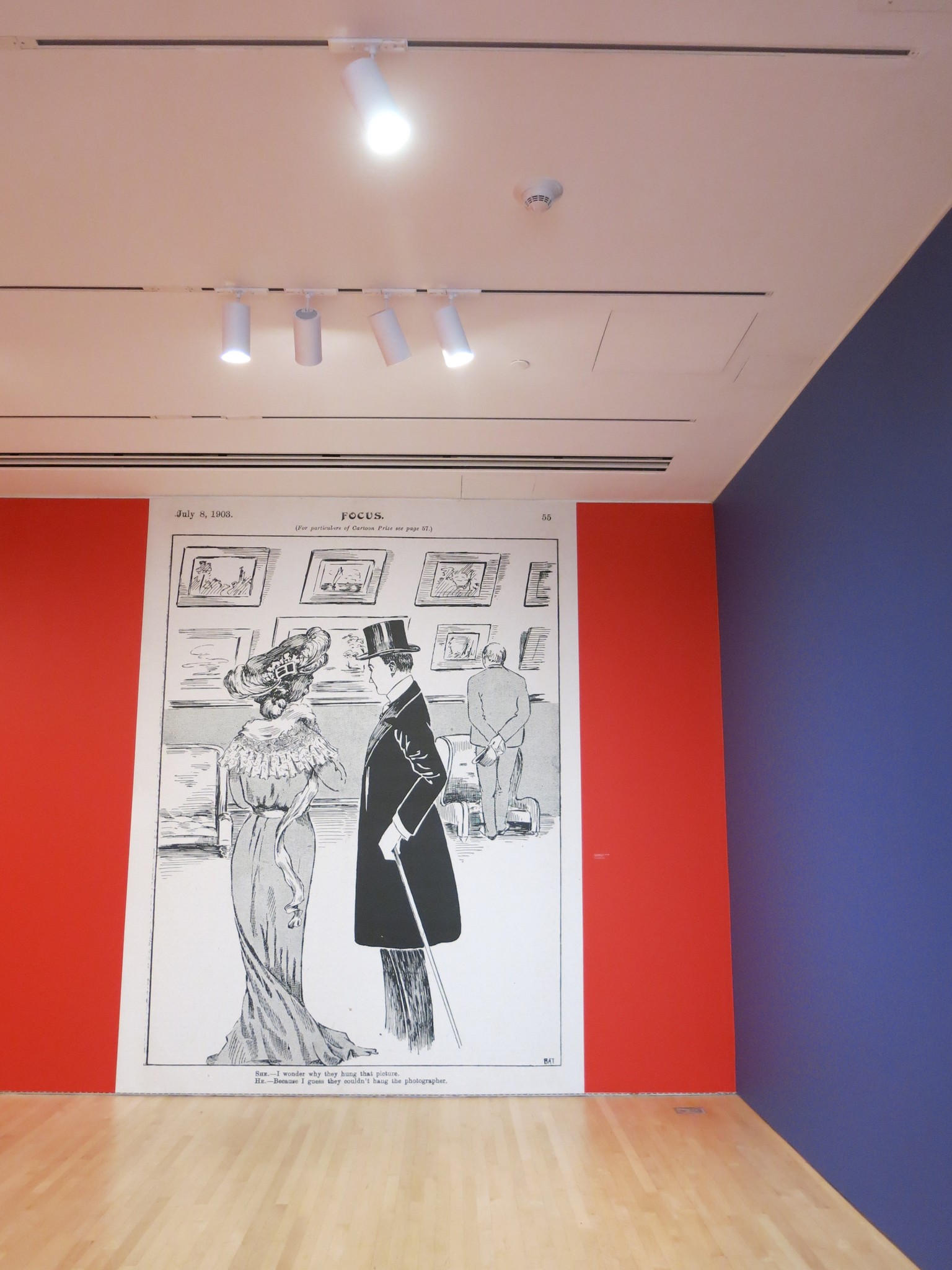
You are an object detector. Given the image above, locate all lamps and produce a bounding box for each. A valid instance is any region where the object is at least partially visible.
[292,298,323,366]
[221,290,251,363]
[345,47,414,160]
[369,295,412,366]
[436,296,474,367]
